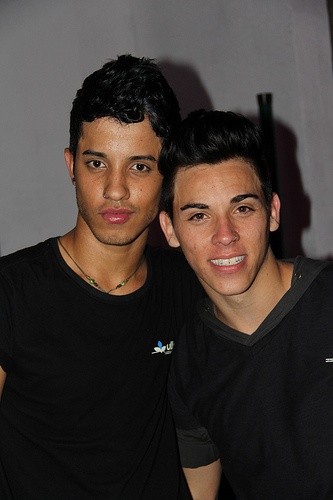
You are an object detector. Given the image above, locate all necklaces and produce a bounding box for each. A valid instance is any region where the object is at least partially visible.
[57,236,150,295]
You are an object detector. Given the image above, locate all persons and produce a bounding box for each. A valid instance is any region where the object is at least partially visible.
[0,54,207,500]
[161,106,333,500]
